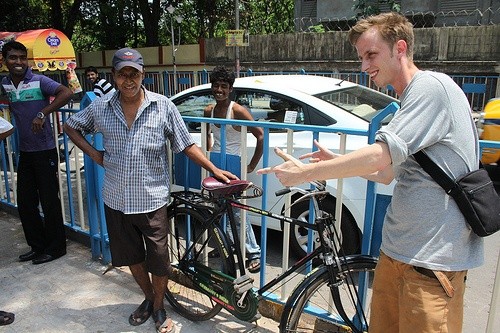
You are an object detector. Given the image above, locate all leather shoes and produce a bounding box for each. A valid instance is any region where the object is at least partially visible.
[32,253,58,263]
[19,250,35,260]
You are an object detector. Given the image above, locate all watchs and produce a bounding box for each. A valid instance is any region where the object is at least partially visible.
[36,112,47,119]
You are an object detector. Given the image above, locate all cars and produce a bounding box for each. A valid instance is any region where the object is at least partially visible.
[163,75,405,269]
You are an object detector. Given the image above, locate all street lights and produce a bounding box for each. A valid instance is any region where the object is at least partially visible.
[161,0,184,88]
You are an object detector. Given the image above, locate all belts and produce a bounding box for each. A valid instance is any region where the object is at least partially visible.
[432,270,455,298]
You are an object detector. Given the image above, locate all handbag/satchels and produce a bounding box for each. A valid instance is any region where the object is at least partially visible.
[451,163,500,237]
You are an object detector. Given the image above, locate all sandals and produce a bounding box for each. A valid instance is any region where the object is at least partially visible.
[208,248,220,258]
[129,300,153,326]
[248,259,261,271]
[152,308,175,333]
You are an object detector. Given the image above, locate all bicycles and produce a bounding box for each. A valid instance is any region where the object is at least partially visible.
[163,176,379,333]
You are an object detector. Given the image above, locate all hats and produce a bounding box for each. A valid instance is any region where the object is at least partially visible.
[112,47,144,72]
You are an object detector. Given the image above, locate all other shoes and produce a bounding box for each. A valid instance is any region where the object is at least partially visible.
[0,310,14,325]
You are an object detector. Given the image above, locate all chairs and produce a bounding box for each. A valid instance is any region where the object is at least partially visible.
[266,96,290,123]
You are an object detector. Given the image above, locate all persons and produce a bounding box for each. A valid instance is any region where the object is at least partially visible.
[205,65,264,272]
[63,48,240,333]
[256,12,483,333]
[0,116,14,325]
[0,42,74,262]
[85,66,116,97]
